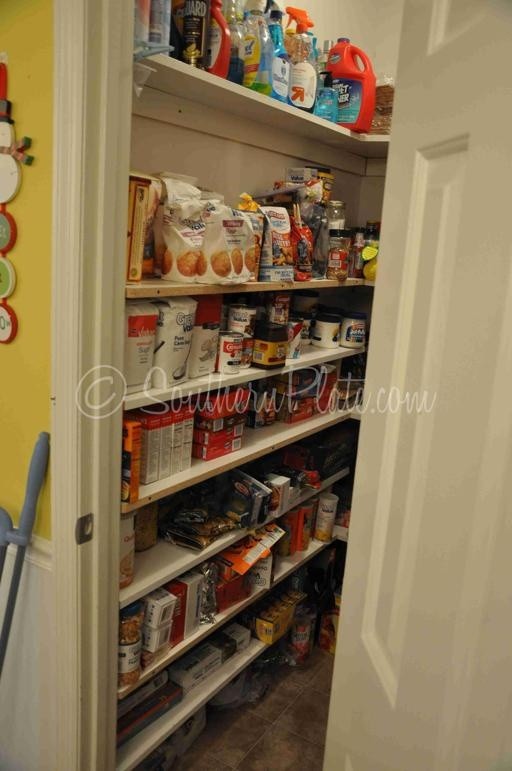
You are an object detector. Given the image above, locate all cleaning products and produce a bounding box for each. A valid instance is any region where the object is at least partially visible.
[205,0,333,122]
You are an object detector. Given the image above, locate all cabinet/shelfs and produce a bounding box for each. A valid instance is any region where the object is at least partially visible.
[1,0,392,771]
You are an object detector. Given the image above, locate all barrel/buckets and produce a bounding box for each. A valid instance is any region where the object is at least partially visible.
[323,38,376,129]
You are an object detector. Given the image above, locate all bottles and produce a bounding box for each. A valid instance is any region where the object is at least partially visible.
[325,200,380,281]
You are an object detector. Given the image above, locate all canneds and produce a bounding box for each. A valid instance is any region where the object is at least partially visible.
[325,229,352,281]
